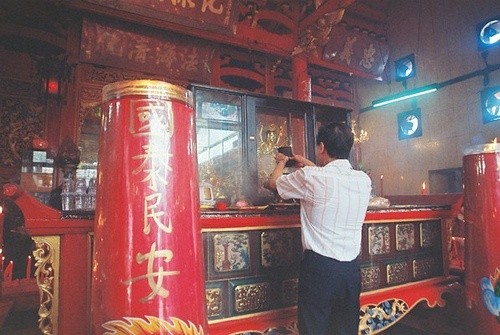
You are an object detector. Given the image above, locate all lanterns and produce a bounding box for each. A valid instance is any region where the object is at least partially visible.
[37,53,77,106]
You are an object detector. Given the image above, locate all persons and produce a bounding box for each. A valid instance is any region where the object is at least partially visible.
[264,119,373,335]
[257,120,293,189]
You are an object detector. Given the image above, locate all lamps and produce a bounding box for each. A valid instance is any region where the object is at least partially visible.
[37,53,72,103]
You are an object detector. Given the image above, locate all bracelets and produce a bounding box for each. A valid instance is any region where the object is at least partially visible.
[277,158,286,169]
